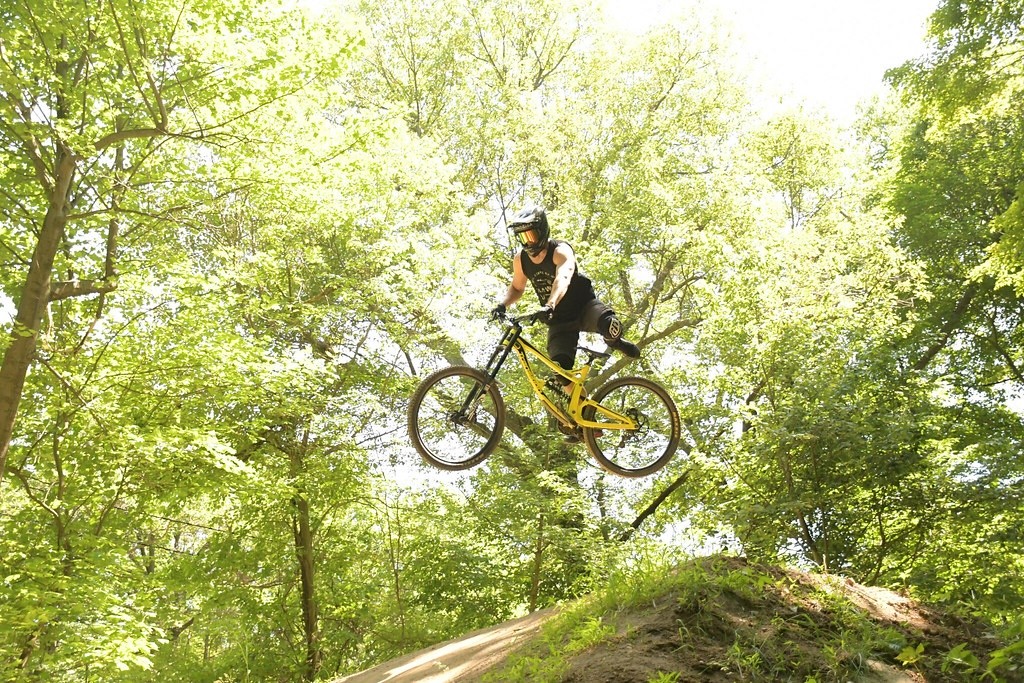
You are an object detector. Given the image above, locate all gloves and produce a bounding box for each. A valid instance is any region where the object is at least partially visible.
[491,303,506,321]
[536,304,554,323]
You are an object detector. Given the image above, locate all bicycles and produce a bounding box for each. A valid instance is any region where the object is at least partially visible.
[408,312,681,478]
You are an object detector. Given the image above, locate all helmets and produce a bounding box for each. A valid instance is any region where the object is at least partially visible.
[507,205,550,257]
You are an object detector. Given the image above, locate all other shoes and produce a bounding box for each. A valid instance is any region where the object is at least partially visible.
[564,421,603,443]
[604,338,640,358]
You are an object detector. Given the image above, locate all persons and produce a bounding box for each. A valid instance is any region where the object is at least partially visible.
[489,207,640,444]
